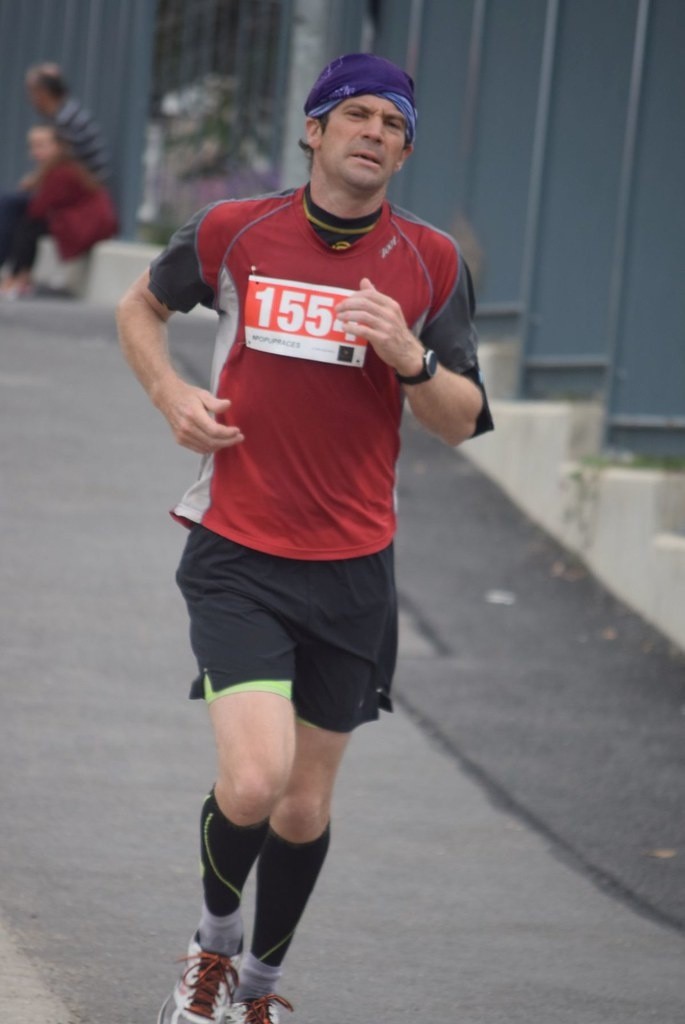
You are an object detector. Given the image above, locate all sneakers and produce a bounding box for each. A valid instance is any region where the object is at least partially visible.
[156,925,244,1024]
[223,985,294,1024]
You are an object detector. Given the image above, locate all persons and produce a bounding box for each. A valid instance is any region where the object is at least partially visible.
[116,54,496,1024]
[0,59,119,273]
[0,126,122,298]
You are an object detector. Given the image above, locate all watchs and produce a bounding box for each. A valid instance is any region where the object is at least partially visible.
[396,348,435,385]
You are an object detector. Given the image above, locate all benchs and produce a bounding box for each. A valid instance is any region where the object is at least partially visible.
[31,235,166,305]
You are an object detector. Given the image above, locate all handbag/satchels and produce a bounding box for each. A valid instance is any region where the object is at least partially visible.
[50,189,116,259]
